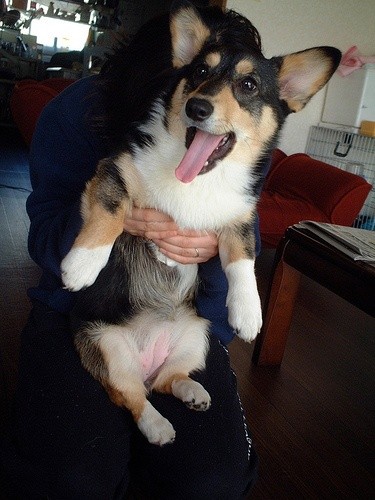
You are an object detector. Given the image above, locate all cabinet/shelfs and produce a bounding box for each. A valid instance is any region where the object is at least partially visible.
[0,0,108,77]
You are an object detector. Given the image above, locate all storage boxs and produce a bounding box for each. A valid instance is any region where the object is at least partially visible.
[319,63,375,133]
[44,67,78,79]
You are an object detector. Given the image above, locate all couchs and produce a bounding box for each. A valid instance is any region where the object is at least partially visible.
[256,149,373,250]
[11,77,76,149]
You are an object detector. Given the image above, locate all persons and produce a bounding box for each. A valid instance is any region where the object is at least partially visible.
[13,0,271,500]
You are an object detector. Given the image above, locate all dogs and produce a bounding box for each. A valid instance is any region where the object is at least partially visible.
[60,0,343,448]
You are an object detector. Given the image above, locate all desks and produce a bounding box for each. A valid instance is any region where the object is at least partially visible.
[250,226,375,369]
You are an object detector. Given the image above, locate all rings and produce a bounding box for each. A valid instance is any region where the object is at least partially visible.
[195,247,199,257]
